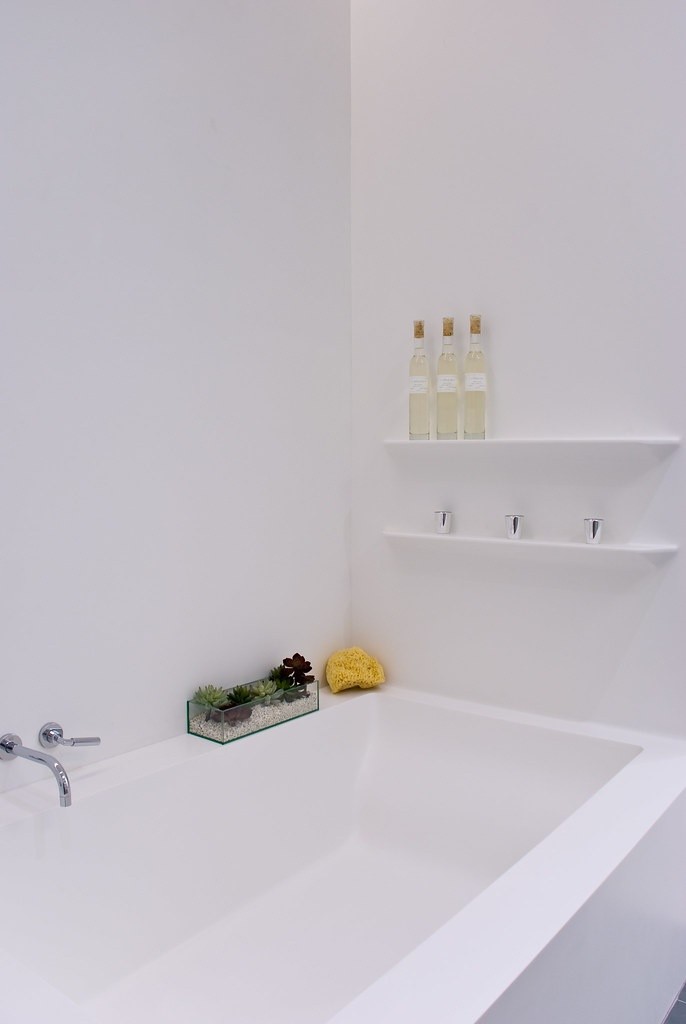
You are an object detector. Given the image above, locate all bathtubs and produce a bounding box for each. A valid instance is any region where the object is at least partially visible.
[0,694,686,1023]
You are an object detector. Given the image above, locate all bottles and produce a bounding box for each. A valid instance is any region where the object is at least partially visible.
[435,317,458,435]
[463,315,487,435]
[407,320,429,441]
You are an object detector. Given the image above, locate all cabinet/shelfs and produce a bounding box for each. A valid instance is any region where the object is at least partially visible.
[377,437,680,554]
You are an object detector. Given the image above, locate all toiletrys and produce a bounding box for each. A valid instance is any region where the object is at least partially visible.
[408,320,430,440]
[465,315,486,440]
[436,316,459,440]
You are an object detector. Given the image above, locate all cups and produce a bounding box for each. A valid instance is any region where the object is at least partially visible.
[584,518,603,544]
[433,510,451,534]
[505,514,524,540]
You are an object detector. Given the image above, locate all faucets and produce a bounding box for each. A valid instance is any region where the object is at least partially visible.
[0,733,72,808]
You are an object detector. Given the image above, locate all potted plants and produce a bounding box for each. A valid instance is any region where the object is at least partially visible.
[187,652,319,744]
